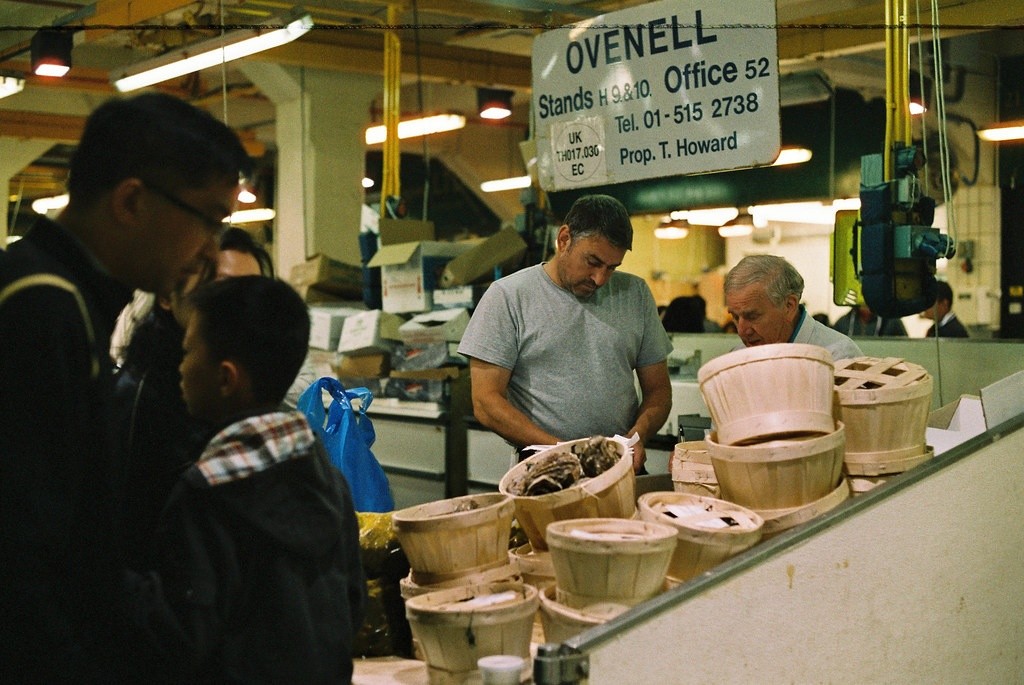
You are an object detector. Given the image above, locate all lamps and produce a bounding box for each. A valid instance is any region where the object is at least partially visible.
[0,14,1024,237]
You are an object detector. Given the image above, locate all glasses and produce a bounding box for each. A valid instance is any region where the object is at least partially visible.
[137,175,230,246]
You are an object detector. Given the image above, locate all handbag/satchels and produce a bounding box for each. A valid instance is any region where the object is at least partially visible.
[298,377,392,515]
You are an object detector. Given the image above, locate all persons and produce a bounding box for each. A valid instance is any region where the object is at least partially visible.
[724,255,866,361]
[0,91,360,685]
[832,305,907,337]
[456,194,674,477]
[657,295,736,333]
[919,281,969,338]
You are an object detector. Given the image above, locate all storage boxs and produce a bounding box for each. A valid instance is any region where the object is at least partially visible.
[286,220,524,397]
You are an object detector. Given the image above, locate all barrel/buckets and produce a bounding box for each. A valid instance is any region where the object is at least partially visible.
[394,344,934,685]
